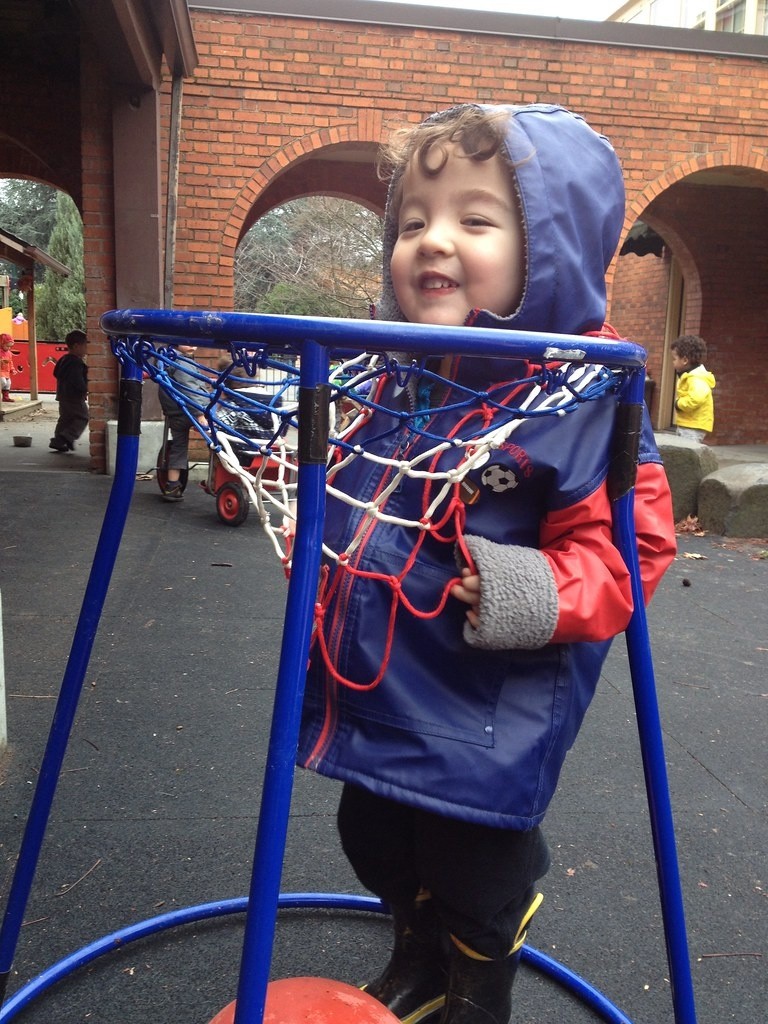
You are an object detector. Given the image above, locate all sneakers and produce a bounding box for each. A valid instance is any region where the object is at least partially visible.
[162,482,185,502]
[49,438,69,452]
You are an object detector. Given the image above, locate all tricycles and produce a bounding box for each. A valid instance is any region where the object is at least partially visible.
[141,370,295,527]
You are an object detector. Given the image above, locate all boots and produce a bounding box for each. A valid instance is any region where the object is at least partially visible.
[2,389,15,402]
[440,892,543,1024]
[356,888,446,1024]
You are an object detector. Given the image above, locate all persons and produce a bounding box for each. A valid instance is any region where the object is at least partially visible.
[296,103,677,1024]
[158,342,210,501]
[671,334,716,444]
[200,353,292,489]
[0,334,17,402]
[48,329,89,452]
